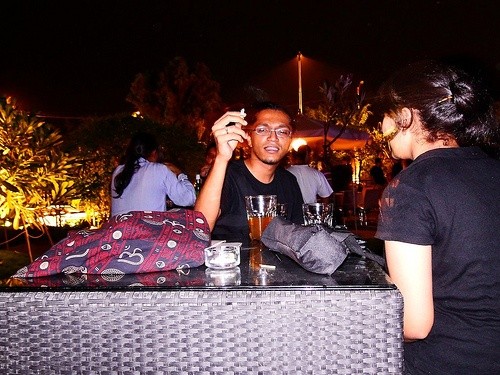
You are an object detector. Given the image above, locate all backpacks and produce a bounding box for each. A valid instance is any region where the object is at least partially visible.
[9,208,212,278]
[5,271,206,287]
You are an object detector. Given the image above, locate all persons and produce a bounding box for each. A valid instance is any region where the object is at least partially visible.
[375,57,500,375]
[110,133,196,216]
[194,102,305,248]
[200,143,413,205]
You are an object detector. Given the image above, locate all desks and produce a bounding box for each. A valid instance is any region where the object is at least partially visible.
[0,240,405,375]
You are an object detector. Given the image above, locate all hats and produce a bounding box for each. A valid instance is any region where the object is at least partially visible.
[343,156,351,161]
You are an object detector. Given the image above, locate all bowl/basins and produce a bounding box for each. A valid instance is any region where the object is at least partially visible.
[204,245,241,270]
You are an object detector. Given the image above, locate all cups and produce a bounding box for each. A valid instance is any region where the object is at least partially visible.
[303,203,333,227]
[245,195,277,241]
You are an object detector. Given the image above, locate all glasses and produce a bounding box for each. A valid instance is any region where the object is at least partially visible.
[252,124,292,139]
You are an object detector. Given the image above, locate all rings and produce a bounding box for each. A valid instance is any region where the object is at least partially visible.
[223,127,228,134]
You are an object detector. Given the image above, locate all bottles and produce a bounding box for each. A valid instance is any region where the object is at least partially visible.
[194,174,202,197]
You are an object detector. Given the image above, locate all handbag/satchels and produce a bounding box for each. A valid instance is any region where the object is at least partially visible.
[270,260,337,287]
[260,215,385,277]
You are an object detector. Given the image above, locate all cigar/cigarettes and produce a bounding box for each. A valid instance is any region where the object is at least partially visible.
[241,107,245,113]
